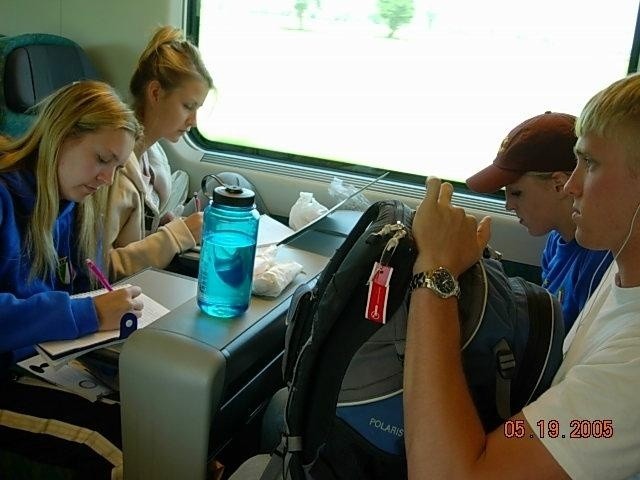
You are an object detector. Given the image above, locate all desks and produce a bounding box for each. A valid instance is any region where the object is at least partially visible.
[77,267,200,394]
[180,244,201,264]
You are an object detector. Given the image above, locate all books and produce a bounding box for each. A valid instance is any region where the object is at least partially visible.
[32,284,172,372]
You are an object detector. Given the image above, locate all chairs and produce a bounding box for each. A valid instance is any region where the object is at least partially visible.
[0,33,100,113]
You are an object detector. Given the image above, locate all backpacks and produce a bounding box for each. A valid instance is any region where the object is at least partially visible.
[262,201,564,480]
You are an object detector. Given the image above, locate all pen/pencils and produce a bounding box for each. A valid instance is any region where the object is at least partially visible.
[193,191,200,213]
[85,259,113,292]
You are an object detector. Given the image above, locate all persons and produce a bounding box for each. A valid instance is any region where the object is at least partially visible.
[465,111,614,338]
[227,73,640,480]
[0,79,145,480]
[104,23,216,285]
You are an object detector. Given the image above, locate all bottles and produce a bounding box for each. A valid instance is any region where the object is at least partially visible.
[196,172,259,320]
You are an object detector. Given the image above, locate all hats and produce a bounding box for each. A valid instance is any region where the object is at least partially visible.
[466,112,579,193]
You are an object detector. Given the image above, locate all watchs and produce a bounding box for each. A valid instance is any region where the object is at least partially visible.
[409,265,461,300]
